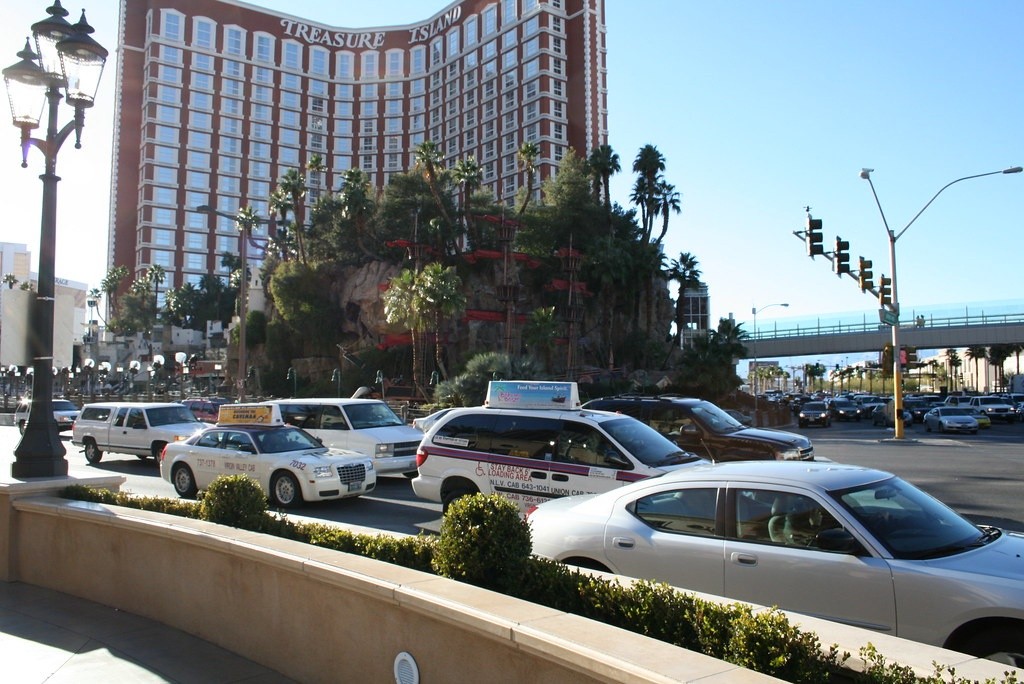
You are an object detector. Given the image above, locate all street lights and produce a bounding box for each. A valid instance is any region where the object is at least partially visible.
[248,365,257,397]
[428,371,440,407]
[375,370,386,401]
[857,166,1024,439]
[0,0,110,480]
[753,302,791,409]
[330,368,342,398]
[195,204,249,402]
[286,367,297,398]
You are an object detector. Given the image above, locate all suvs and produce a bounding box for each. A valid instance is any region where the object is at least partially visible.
[798,400,832,428]
[262,396,427,479]
[580,390,815,463]
[13,398,81,436]
[71,401,209,464]
[181,396,233,425]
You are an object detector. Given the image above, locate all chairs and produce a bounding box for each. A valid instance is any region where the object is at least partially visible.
[228,435,246,450]
[768,493,795,542]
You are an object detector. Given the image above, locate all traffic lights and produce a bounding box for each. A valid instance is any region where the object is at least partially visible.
[815,362,820,373]
[805,213,824,258]
[878,273,893,308]
[833,236,851,278]
[900,345,918,374]
[882,342,895,374]
[835,364,839,372]
[857,256,874,294]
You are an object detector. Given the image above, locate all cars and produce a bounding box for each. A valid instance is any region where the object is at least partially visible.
[522,458,1023,670]
[761,389,1024,429]
[922,406,979,435]
[410,378,713,522]
[159,401,377,510]
[412,405,458,434]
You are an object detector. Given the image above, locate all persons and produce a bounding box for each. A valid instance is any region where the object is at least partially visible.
[784,499,826,546]
